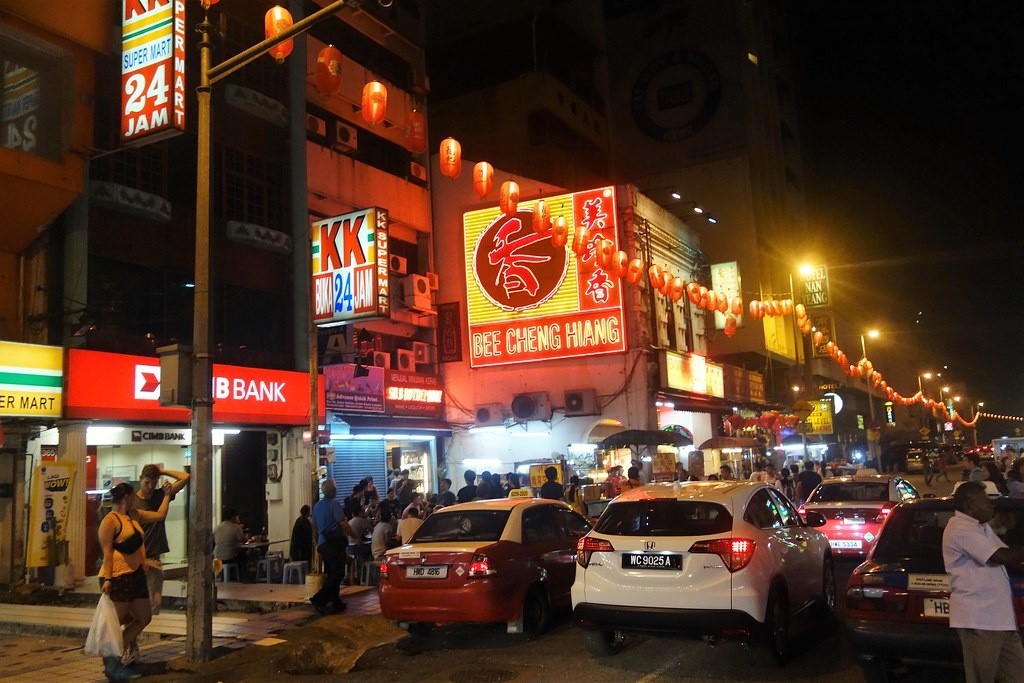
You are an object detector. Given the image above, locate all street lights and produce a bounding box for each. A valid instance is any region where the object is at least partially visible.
[940,386,950,403]
[918,371,931,428]
[789,262,813,462]
[861,330,884,475]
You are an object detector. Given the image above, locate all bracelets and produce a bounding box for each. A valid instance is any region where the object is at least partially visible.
[105,579,112,582]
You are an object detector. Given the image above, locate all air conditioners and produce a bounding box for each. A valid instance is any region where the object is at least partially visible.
[308,114,327,136]
[408,161,426,181]
[411,342,430,364]
[510,391,548,423]
[331,121,358,151]
[397,349,417,374]
[564,387,599,417]
[371,349,390,370]
[427,271,440,292]
[387,254,408,274]
[475,402,503,426]
[405,273,431,308]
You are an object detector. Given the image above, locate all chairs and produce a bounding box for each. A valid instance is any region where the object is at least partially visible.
[908,525,944,555]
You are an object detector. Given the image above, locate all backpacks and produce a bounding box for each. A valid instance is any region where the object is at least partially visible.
[784,483,794,502]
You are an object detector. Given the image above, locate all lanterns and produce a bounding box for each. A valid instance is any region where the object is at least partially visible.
[201,0,219,8]
[265,5,293,63]
[362,80,388,127]
[316,45,343,103]
[405,109,428,156]
[440,137,1024,430]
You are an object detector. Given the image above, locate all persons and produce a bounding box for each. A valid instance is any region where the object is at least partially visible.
[129,464,189,660]
[920,446,1024,497]
[289,480,361,615]
[341,468,520,585]
[541,467,584,513]
[708,464,737,480]
[943,482,1024,683]
[98,480,173,683]
[750,454,840,508]
[212,510,247,581]
[605,465,643,498]
[674,462,699,481]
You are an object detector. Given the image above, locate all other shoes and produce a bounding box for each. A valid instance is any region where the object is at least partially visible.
[123,644,140,663]
[101,658,143,680]
[308,592,345,614]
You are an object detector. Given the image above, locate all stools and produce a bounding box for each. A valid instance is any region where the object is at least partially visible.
[219,550,310,584]
[342,544,389,587]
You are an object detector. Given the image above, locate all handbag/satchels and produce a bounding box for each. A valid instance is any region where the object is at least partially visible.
[84,591,123,658]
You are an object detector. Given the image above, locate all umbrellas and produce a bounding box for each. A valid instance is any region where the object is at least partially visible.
[601,430,693,461]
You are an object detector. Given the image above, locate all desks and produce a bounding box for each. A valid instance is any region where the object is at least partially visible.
[238,539,290,580]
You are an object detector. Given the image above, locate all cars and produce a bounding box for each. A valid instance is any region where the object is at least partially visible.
[844,480,1024,683]
[798,475,921,556]
[573,480,838,669]
[379,498,603,637]
[968,445,993,460]
[904,440,964,474]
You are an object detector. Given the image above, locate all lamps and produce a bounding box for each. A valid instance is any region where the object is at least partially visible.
[69,321,95,336]
[354,362,370,378]
[680,210,718,226]
[656,199,705,218]
[639,184,679,204]
[358,326,374,344]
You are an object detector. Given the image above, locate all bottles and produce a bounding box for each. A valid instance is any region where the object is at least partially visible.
[101,494,112,519]
[261,526,266,543]
